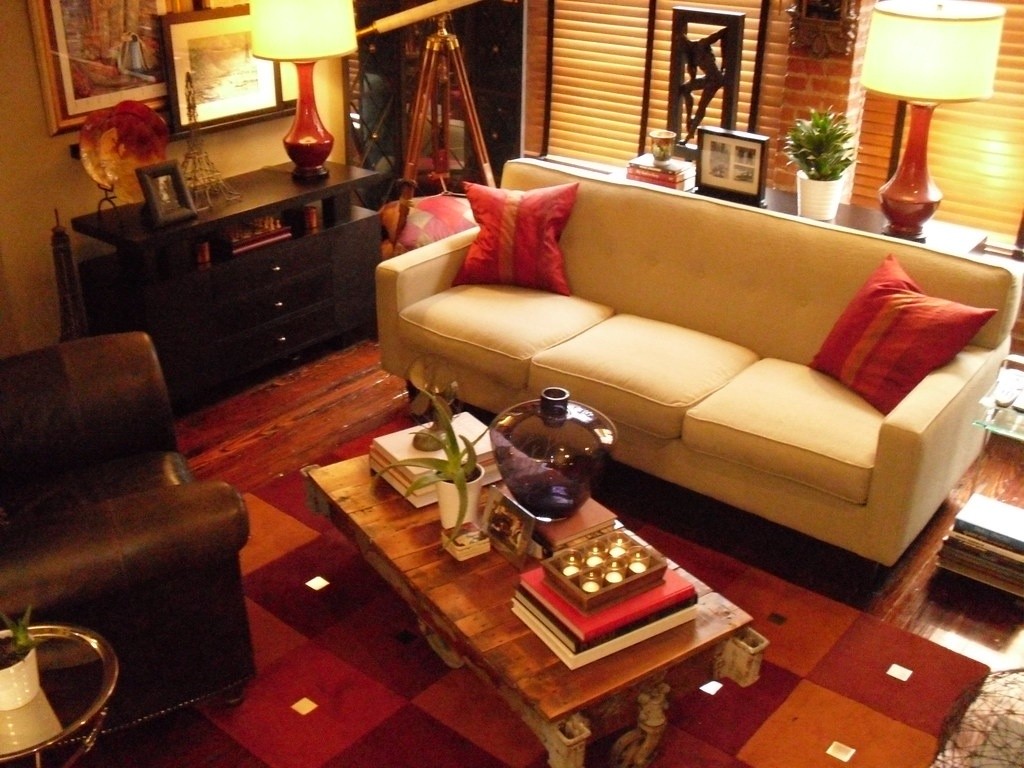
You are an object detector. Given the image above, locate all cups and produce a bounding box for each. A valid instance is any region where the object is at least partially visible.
[649,130,676,166]
[559,533,650,593]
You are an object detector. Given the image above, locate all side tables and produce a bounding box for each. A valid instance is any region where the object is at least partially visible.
[1,623,118,768]
[971,353,1024,503]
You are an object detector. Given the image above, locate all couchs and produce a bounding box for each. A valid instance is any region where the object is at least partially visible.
[376,159,1024,581]
[0,331,255,750]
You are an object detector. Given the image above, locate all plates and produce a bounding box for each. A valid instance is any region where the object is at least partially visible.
[79,101,169,202]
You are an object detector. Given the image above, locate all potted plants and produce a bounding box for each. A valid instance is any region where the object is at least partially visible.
[785,106,856,220]
[374,387,490,553]
[0,605,65,756]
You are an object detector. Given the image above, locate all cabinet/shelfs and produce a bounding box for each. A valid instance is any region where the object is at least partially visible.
[71,160,382,422]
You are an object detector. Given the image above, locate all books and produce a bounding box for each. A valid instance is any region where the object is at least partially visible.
[627,154,695,191]
[229,227,292,254]
[370,411,699,670]
[936,492,1024,598]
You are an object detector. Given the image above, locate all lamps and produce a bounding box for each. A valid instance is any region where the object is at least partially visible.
[251,0,358,183]
[860,0,1008,243]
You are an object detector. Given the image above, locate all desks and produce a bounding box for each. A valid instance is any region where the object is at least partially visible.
[605,167,987,256]
[308,456,769,767]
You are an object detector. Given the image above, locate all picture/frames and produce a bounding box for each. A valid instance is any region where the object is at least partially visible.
[26,0,212,137]
[134,158,199,230]
[696,126,771,209]
[479,485,536,572]
[161,3,284,135]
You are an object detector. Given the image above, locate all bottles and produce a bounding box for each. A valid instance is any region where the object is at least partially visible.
[194,237,211,264]
[490,387,618,520]
[303,205,318,230]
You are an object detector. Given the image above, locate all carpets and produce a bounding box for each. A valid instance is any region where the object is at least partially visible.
[83,412,991,768]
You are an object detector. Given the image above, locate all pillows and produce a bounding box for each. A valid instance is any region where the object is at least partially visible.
[377,190,478,258]
[451,181,579,297]
[806,253,999,418]
